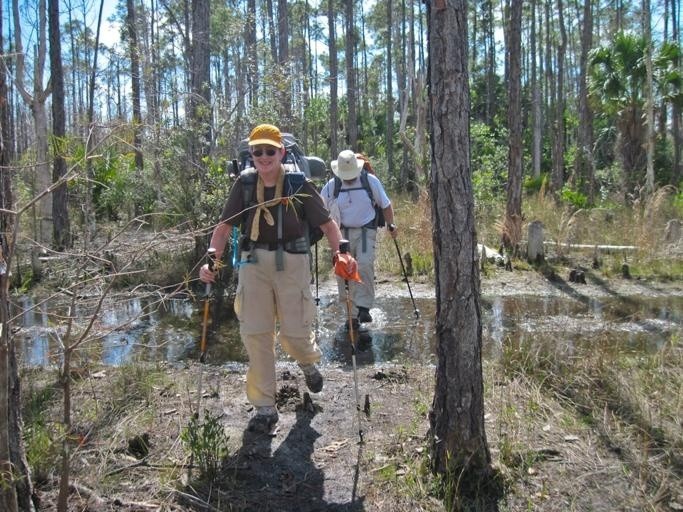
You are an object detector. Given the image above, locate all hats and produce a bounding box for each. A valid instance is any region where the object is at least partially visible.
[249,123,284,150]
[330,149,366,181]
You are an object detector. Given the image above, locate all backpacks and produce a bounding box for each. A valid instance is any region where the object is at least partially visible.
[225,133,325,248]
[333,153,385,228]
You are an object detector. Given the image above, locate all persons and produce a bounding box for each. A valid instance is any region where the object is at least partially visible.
[320,150,398,329]
[197,124,356,431]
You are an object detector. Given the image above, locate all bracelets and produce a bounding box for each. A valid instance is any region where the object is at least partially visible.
[388,223,395,226]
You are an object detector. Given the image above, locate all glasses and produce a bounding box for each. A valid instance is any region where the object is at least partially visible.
[251,148,276,157]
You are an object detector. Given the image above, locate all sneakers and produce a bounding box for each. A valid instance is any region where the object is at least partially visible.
[248,412,279,431]
[344,306,373,329]
[300,367,325,393]
